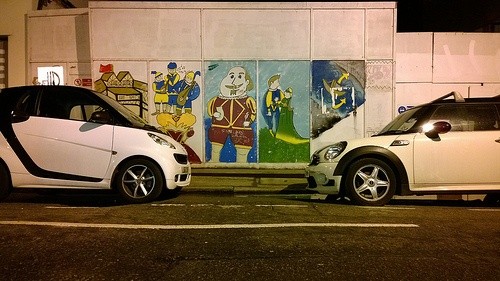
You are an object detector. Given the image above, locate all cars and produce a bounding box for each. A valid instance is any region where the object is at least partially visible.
[1,85,191,202]
[304,91,500,207]
